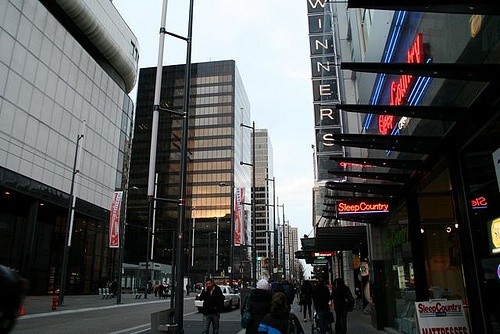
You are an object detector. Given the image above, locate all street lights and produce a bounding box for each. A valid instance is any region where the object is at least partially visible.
[115,187,138,304]
[219,182,234,286]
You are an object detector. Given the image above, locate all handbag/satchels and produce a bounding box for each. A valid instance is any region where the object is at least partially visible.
[241,307,251,328]
[345,298,353,312]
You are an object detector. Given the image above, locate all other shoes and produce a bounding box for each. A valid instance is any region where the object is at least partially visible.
[304,319,307,322]
[309,318,312,320]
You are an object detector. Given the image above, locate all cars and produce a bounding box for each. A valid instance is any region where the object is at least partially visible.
[194,284,241,311]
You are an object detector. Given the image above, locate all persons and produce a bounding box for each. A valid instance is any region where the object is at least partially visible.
[0,265,26,334]
[104,277,313,321]
[331,277,353,333]
[257,292,306,333]
[246,278,273,325]
[200,277,224,334]
[311,280,332,333]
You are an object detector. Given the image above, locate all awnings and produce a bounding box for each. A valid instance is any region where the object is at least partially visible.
[295,225,367,266]
